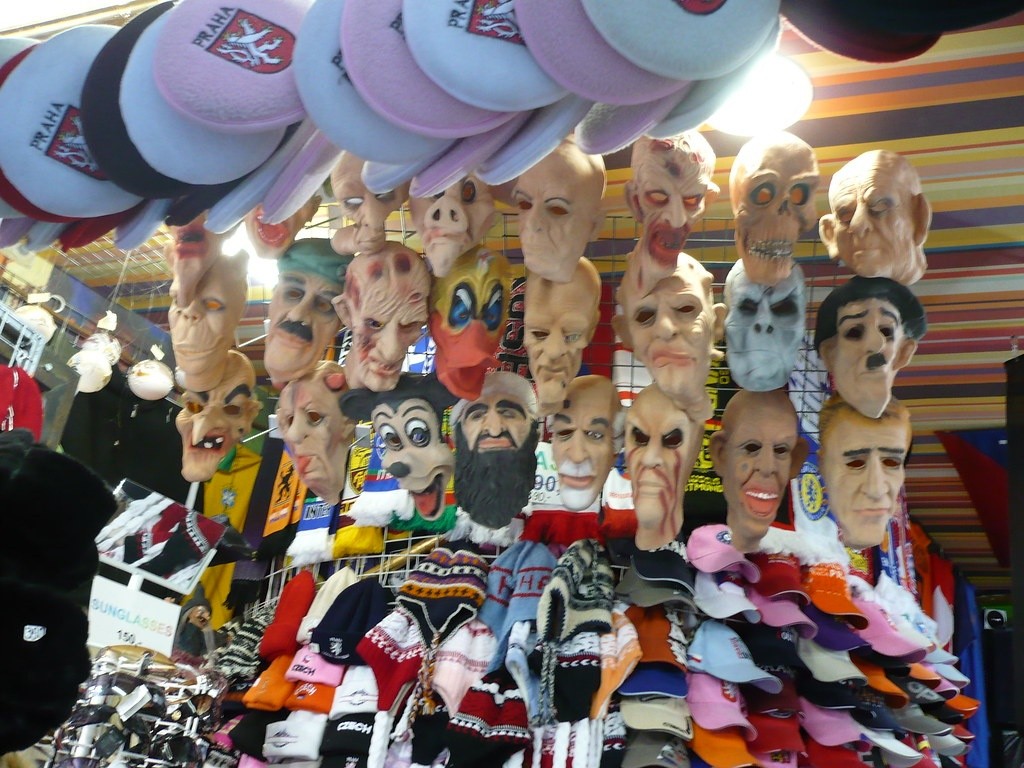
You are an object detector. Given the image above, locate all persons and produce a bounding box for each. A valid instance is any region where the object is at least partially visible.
[169,132,932,552]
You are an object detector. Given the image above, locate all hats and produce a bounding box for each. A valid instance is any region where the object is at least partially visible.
[212,524,981,768]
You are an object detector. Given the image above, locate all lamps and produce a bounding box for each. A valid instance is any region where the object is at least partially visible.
[15,303,59,344]
[66,251,133,392]
[128,358,175,402]
